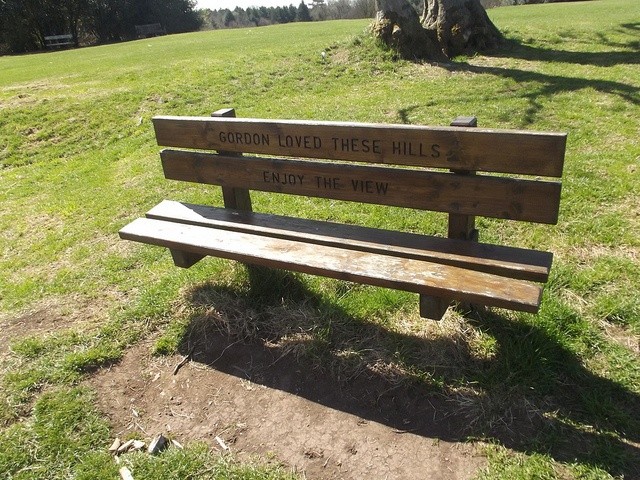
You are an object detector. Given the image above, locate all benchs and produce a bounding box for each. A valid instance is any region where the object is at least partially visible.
[117,107,568,321]
